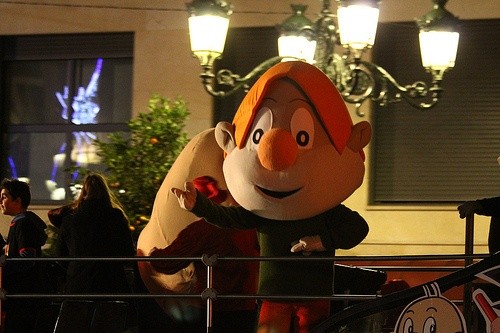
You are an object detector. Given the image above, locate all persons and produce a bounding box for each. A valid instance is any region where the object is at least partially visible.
[457,155,500,333]
[0,176,47,333]
[58,174,136,333]
[151,176,261,333]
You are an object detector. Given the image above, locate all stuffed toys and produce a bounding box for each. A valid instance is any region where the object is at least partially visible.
[170,61,372,333]
